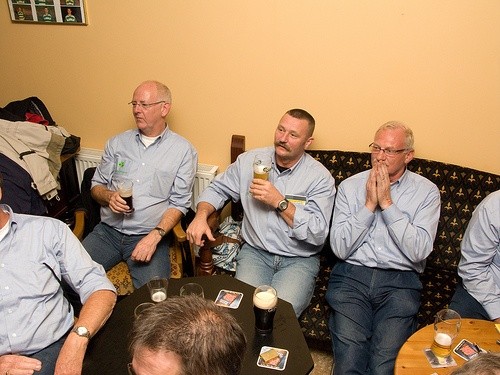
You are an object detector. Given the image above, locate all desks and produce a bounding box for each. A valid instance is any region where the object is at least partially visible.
[393,318,500,375]
[80,274,315,375]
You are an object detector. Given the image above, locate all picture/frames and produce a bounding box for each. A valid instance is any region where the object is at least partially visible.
[6,0,89,26]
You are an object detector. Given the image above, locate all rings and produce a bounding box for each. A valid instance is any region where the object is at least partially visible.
[6,372,8,375]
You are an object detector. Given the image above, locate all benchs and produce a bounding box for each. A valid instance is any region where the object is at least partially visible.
[197,135,500,344]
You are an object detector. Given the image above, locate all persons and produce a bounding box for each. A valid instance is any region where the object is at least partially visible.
[0,186,117,375]
[450,188,500,324]
[186,109,340,319]
[128,296,245,375]
[324,120,441,375]
[77,80,198,289]
[454,354,500,375]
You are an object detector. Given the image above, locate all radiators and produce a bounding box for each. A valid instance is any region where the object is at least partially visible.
[74,146,219,215]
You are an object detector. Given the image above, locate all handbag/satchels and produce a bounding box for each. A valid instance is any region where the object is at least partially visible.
[212,215,244,271]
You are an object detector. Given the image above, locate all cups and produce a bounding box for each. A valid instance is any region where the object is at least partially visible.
[117,181,135,215]
[252,284,278,336]
[180,283,204,299]
[147,277,169,305]
[430,309,462,359]
[252,154,272,201]
[134,303,155,319]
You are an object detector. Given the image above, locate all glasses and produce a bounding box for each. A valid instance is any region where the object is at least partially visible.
[129,101,167,109]
[369,142,408,155]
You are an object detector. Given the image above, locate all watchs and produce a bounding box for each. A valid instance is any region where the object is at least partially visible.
[153,227,165,237]
[275,198,289,213]
[71,327,91,340]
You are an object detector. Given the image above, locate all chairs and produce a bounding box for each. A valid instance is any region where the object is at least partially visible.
[21,96,76,230]
[71,167,188,302]
